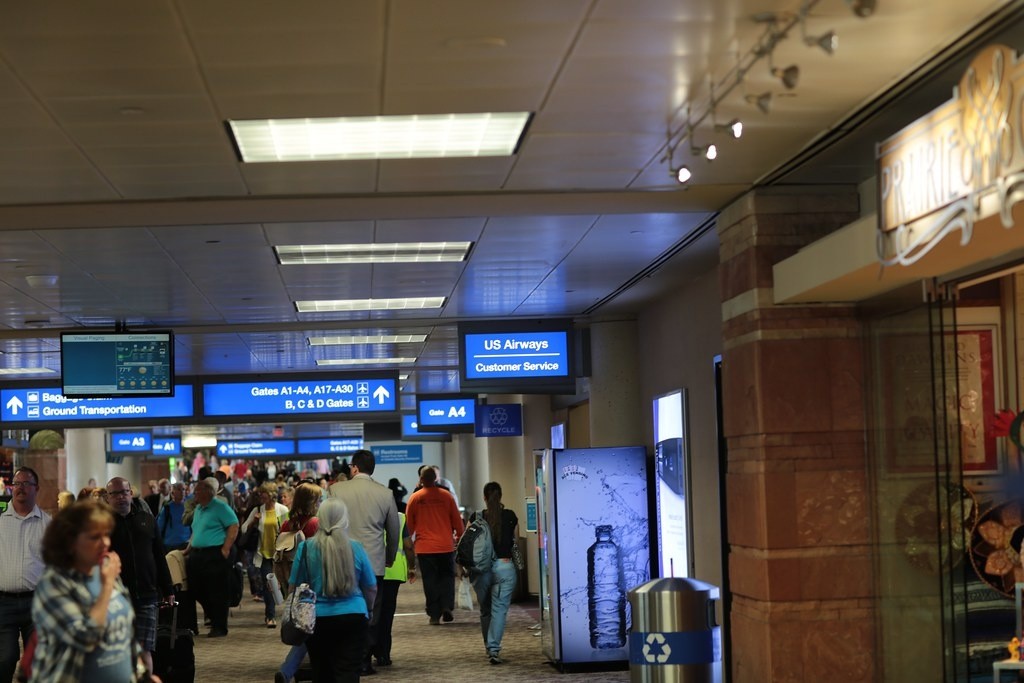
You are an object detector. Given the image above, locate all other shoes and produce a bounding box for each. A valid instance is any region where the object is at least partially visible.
[267,616,276,628]
[361,663,376,676]
[209,630,228,638]
[376,656,392,666]
[274,670,288,683]
[430,616,439,625]
[205,619,211,625]
[441,606,453,622]
[490,654,501,663]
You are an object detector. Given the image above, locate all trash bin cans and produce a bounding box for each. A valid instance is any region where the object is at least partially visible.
[627,577,720,683]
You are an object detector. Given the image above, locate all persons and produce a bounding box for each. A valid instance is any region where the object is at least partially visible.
[459,481,519,664]
[0,448,465,683]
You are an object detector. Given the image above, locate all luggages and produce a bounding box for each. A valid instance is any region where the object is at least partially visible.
[149,600,195,683]
[177,601,199,636]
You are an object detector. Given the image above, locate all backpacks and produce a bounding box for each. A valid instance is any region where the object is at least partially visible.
[457,510,497,577]
[272,517,318,599]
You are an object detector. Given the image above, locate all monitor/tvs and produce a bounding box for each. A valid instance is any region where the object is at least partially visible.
[60,329,175,397]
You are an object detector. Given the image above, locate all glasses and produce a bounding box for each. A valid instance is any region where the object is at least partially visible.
[348,463,356,469]
[107,490,130,497]
[9,481,37,488]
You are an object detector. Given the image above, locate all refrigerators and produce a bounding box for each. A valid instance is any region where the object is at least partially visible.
[534,446,650,672]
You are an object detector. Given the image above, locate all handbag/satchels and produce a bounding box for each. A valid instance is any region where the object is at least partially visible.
[21,622,38,678]
[239,504,261,552]
[458,567,474,612]
[281,582,316,646]
[510,511,524,571]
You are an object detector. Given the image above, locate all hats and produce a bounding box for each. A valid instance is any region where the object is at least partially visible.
[202,477,219,495]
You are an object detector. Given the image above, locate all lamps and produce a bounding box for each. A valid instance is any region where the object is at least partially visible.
[660,0,877,185]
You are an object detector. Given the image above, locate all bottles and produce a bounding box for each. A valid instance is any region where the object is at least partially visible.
[587,526,627,649]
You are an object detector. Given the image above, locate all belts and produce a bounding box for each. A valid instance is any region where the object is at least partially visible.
[191,545,223,552]
